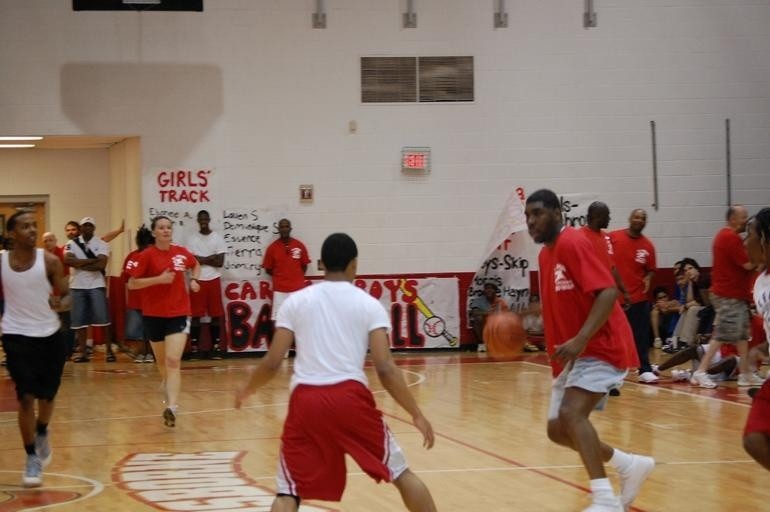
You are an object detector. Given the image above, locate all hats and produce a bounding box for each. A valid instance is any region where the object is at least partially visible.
[79,217,96,229]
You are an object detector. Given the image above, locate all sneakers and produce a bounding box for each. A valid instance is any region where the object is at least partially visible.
[525,342,539,352]
[190,349,201,361]
[209,349,225,360]
[690,370,717,389]
[477,343,488,352]
[579,487,625,512]
[65,343,97,363]
[634,363,662,384]
[163,406,176,428]
[133,352,155,363]
[671,369,692,382]
[23,453,43,487]
[620,451,655,503]
[737,372,766,386]
[106,350,115,363]
[653,337,685,354]
[34,429,52,466]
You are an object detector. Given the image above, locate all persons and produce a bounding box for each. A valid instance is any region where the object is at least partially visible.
[127,215,202,428]
[230,232,438,511]
[261,218,313,360]
[467,256,770,384]
[689,203,770,391]
[0,210,73,487]
[609,208,661,384]
[574,201,633,398]
[523,189,658,511]
[1,209,229,365]
[739,207,770,471]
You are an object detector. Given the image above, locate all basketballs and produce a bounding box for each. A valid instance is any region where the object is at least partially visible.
[481,314,524,358]
